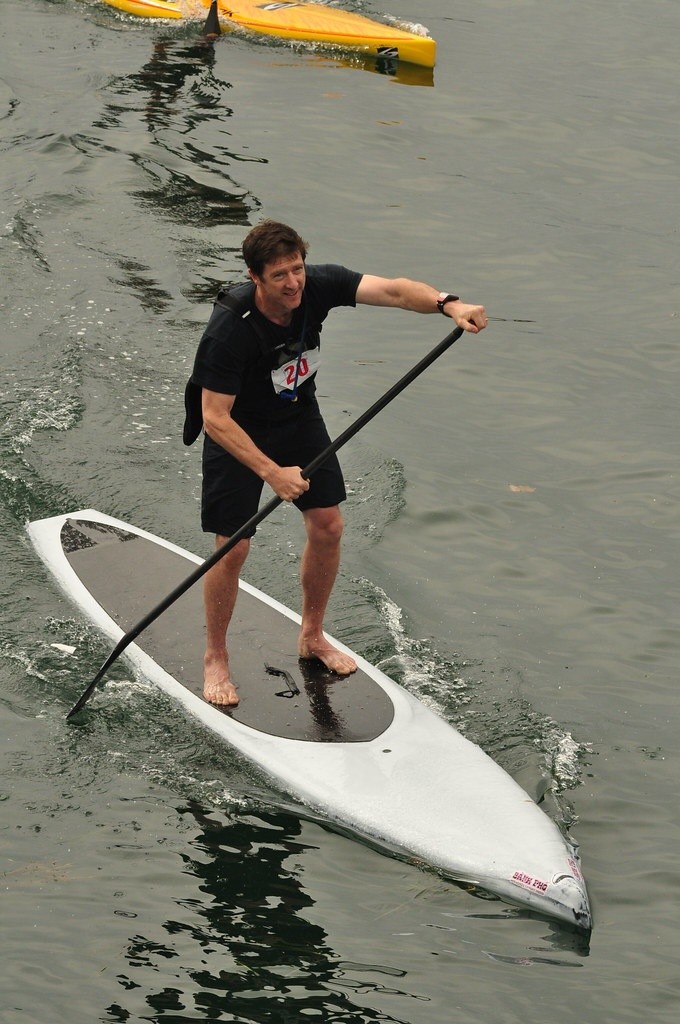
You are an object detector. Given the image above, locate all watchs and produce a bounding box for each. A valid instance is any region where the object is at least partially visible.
[437,291,459,318]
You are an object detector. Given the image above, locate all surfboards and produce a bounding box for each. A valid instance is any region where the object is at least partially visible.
[18,505,594,929]
[105,0,435,71]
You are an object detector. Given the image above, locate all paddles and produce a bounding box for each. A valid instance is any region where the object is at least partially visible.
[204,0,221,35]
[66,324,464,718]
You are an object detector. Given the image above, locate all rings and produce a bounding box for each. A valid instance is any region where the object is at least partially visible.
[486,319,488,320]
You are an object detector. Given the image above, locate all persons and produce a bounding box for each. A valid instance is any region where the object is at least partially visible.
[183,220,488,704]
